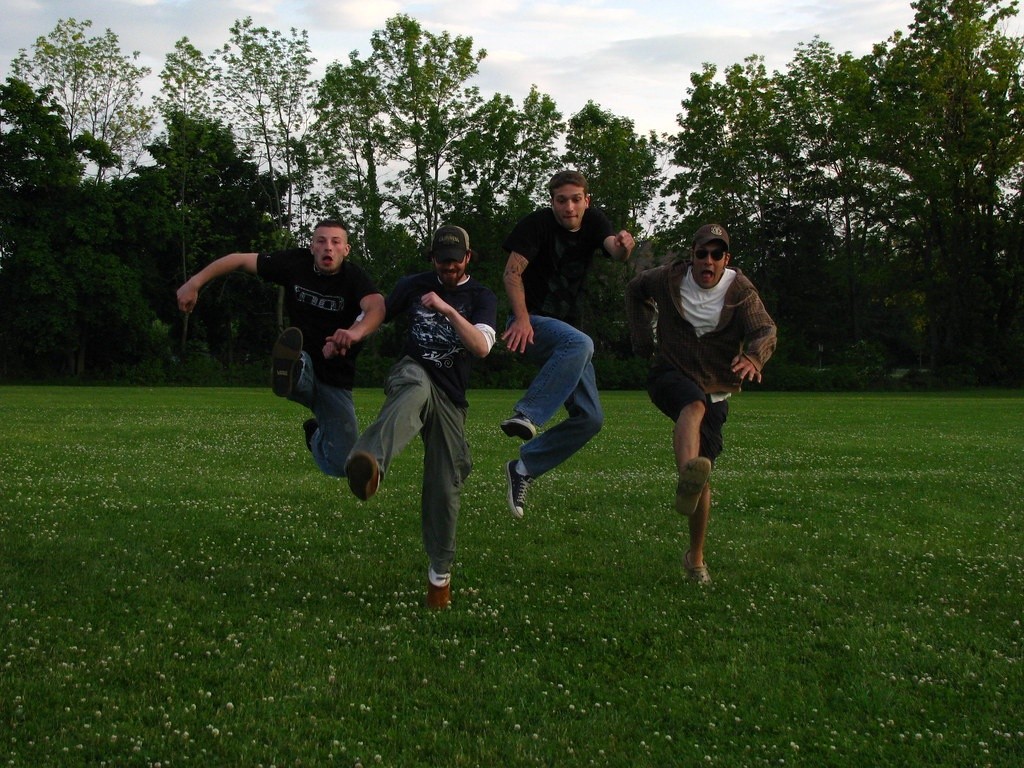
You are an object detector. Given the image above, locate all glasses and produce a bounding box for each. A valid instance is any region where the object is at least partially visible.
[434,256,463,265]
[693,248,727,261]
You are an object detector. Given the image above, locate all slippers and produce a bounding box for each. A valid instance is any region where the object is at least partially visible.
[676,457,711,515]
[683,548,711,584]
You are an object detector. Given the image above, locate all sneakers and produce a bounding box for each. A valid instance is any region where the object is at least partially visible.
[507,459,535,519]
[500,412,536,440]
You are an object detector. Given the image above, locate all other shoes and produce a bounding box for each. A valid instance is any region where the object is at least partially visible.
[303,418,319,453]
[270,327,303,395]
[346,450,378,502]
[427,564,451,609]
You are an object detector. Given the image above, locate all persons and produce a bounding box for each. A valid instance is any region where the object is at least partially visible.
[177,219,385,477]
[624,224,777,583]
[501,171,635,519]
[322,225,498,610]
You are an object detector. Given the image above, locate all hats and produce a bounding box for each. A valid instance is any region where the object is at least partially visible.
[693,223,730,253]
[431,224,469,260]
